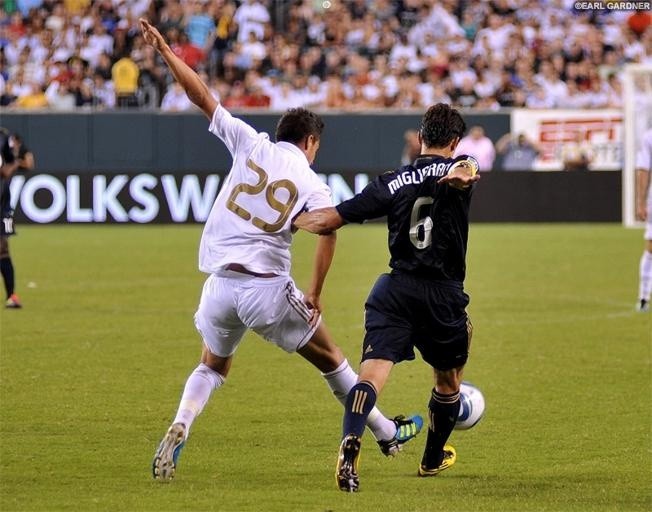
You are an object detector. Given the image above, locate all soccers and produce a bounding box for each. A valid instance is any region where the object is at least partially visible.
[452,383,484,430]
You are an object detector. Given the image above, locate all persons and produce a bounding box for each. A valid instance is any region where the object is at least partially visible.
[496,133,539,171]
[8,135,35,170]
[402,129,421,164]
[129,12,426,481]
[0,0,652,110]
[453,125,496,172]
[0,125,15,311]
[634,128,651,313]
[563,133,595,172]
[291,102,482,491]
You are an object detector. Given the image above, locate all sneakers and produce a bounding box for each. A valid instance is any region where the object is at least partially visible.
[635,300,650,313]
[147,421,191,483]
[5,294,22,309]
[418,444,458,478]
[334,430,364,495]
[376,415,428,457]
